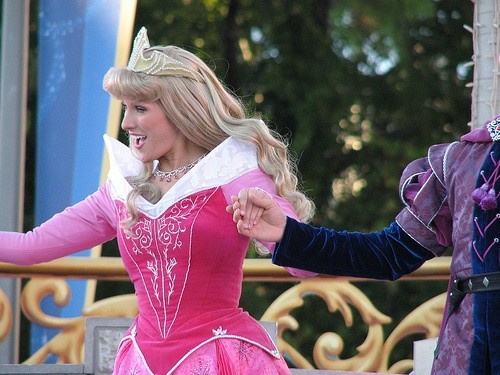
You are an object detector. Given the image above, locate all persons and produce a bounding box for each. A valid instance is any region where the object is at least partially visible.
[2,26,322,375]
[224,113,500,375]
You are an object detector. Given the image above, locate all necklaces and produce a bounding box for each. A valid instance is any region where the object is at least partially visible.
[152,150,209,182]
